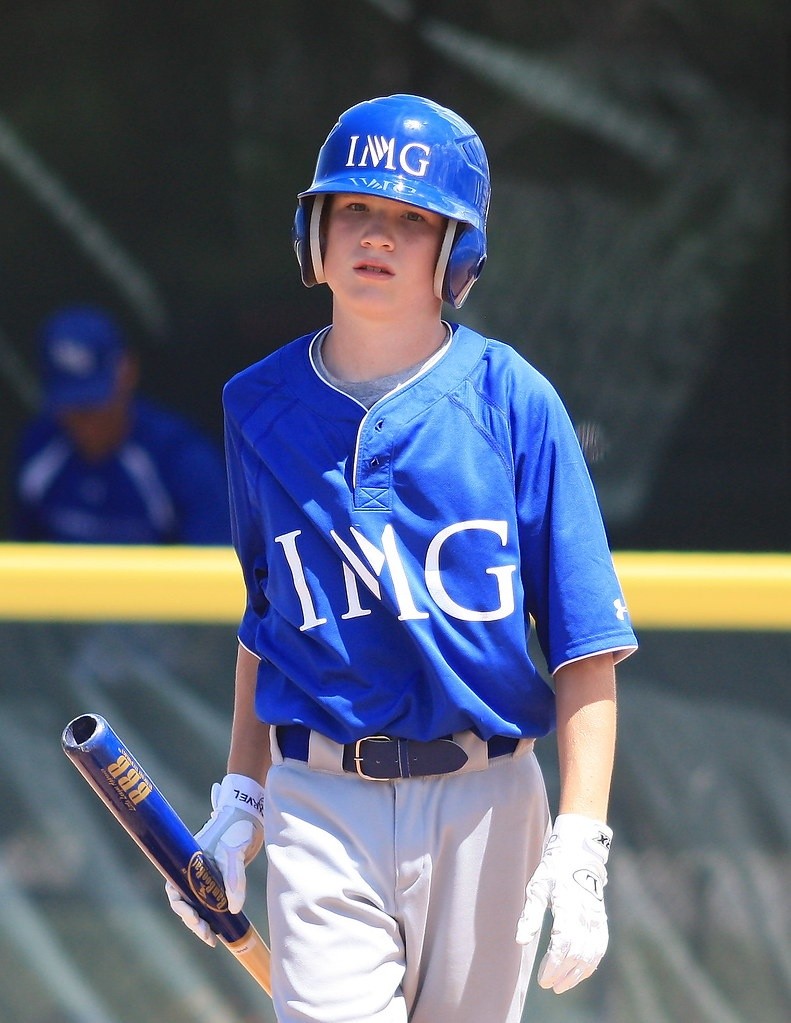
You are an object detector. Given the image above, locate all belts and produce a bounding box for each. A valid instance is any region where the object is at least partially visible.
[279,723,521,778]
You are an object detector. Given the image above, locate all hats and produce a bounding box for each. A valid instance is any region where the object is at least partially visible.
[39,306,127,407]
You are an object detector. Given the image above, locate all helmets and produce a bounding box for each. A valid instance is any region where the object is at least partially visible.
[288,94,490,307]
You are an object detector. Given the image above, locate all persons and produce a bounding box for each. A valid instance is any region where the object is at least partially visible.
[163,94,643,1023]
[9,306,237,547]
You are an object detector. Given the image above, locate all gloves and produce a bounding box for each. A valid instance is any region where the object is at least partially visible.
[514,814,614,994]
[163,774,265,948]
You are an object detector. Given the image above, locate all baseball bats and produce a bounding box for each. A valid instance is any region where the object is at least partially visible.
[58,712,274,1005]
